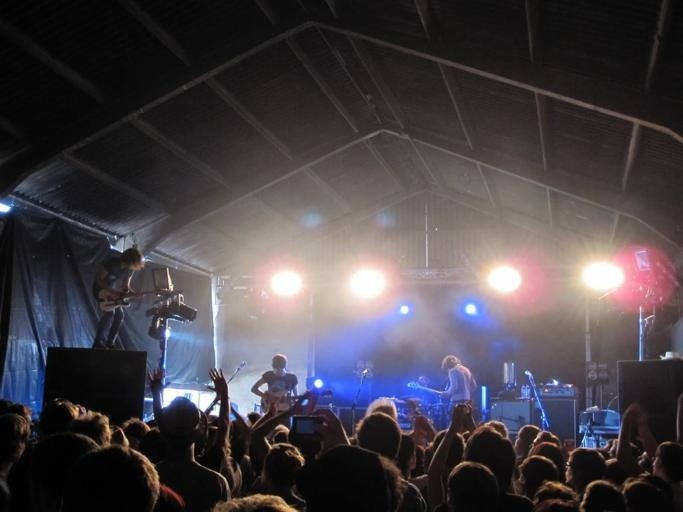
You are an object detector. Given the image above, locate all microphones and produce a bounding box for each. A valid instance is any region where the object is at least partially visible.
[361,368,370,376]
[525,370,532,376]
[238,360,247,369]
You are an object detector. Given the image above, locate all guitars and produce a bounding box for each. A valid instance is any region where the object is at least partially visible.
[261,390,332,413]
[97,288,166,312]
[407,382,451,402]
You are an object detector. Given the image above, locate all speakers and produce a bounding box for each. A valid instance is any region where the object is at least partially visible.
[616,358,683,445]
[38,346,147,440]
[531,398,578,448]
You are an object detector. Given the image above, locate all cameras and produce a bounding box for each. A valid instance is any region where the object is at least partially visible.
[462,406,471,415]
[292,415,325,440]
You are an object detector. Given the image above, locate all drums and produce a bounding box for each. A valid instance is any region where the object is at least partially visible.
[430,403,451,422]
[397,406,409,419]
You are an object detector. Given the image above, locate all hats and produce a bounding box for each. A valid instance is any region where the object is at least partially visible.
[297,445,394,512]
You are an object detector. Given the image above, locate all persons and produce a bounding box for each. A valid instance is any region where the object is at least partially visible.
[92,249,146,349]
[1,367,682,512]
[250,354,299,415]
[440,355,477,424]
